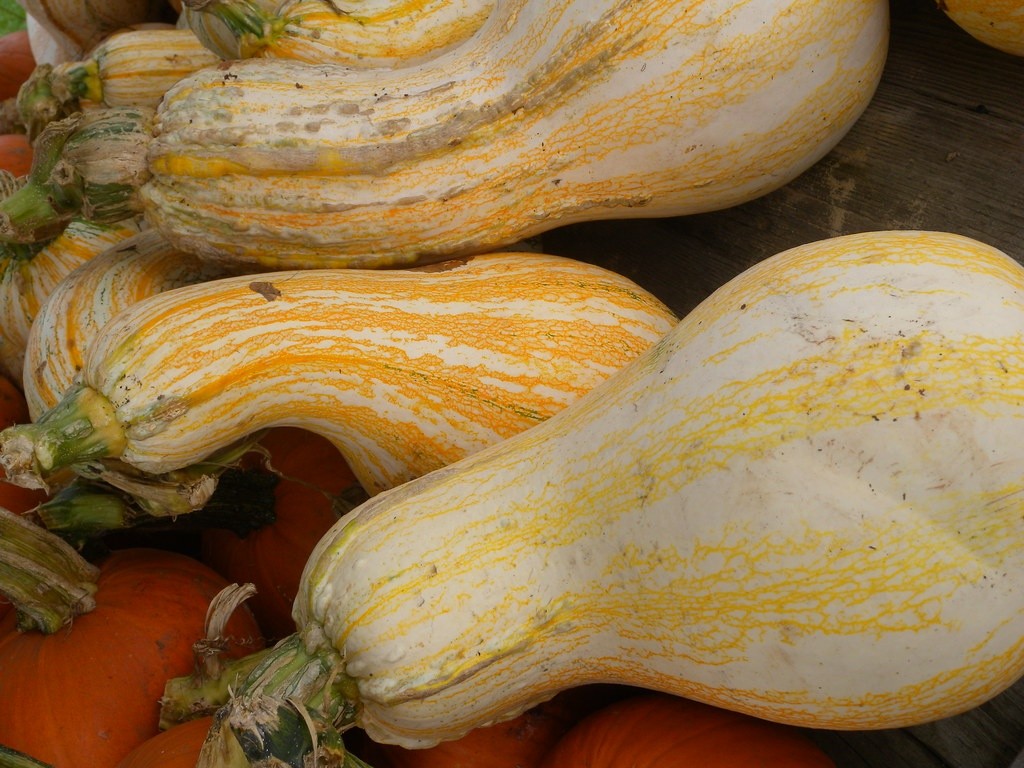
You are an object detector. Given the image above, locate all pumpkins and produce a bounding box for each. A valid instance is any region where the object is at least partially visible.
[0,0,1024,768]
